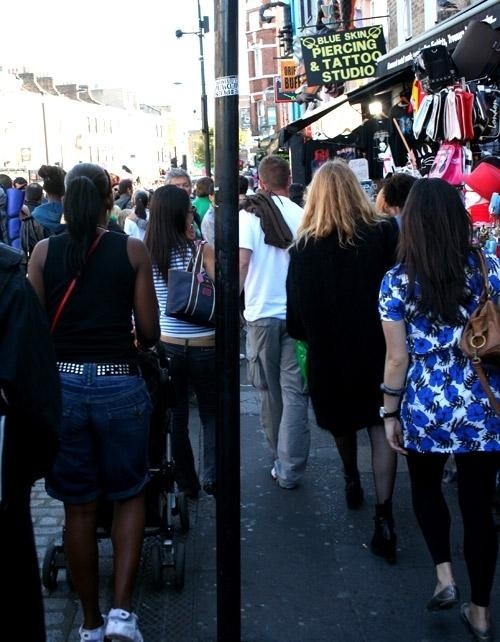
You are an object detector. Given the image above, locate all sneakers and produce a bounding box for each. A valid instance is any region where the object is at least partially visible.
[105,607,143,641]
[78,614,108,642]
[270,466,298,488]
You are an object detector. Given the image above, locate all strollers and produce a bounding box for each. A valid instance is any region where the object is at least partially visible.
[36,344,196,600]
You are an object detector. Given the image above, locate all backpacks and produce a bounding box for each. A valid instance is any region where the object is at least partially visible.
[413,19,499,184]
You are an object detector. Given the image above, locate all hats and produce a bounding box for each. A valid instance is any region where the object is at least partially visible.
[458,162,500,201]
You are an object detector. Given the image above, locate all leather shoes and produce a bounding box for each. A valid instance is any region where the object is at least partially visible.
[461,603,493,638]
[426,584,461,612]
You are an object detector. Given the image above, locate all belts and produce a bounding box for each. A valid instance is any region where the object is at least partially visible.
[159,335,217,347]
[52,361,135,375]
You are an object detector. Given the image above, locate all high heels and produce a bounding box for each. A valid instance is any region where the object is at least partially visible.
[370,499,397,562]
[344,474,364,510]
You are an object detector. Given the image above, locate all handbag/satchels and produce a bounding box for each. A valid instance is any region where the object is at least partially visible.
[462,245,499,415]
[166,239,216,328]
[21,216,54,259]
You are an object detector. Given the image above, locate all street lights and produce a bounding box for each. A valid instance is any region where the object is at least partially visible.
[173,0,212,177]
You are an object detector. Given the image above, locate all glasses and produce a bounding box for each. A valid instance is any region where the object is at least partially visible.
[462,185,475,196]
